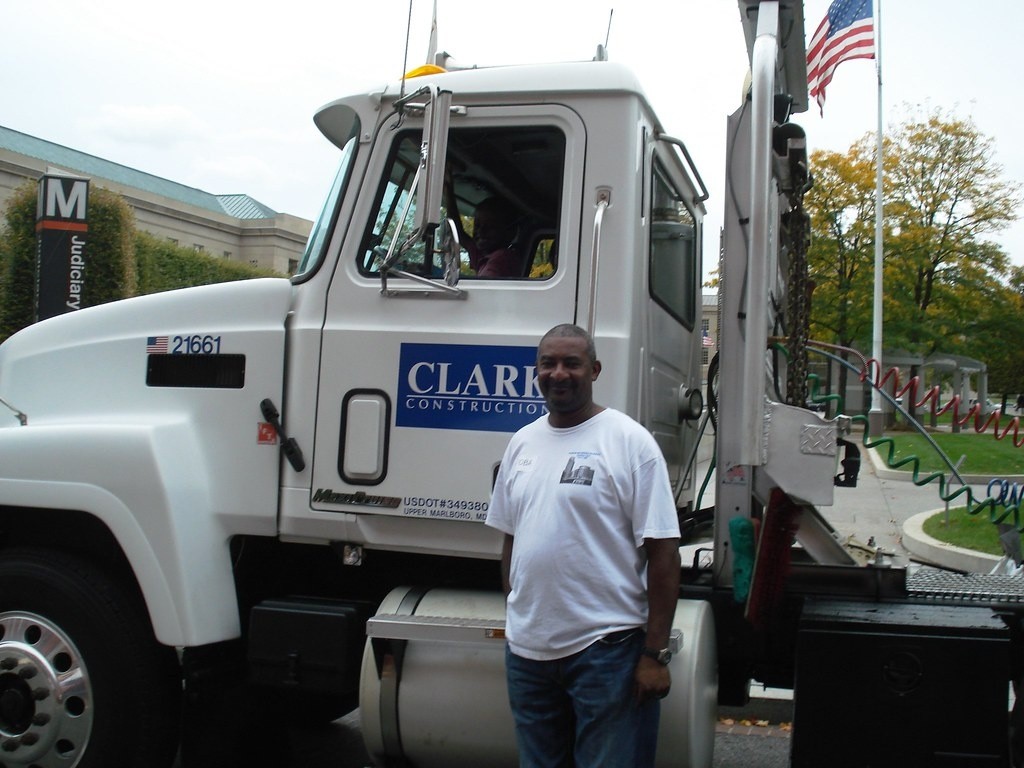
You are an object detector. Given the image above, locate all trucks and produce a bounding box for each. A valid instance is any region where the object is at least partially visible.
[0,1,1024,768]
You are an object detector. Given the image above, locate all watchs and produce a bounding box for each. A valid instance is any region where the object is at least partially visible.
[642,645,674,667]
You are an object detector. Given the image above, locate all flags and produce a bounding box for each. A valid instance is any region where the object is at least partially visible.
[806,0,875,116]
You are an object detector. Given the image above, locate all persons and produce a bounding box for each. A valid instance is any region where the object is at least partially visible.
[485,322,683,767]
[445,164,523,279]
